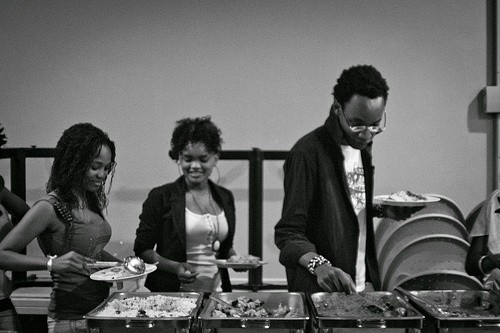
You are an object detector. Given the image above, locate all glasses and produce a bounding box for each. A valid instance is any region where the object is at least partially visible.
[342,108,386,133]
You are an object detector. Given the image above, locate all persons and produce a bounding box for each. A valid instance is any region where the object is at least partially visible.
[274,64,426,294]
[0,183,31,333]
[464,189,500,276]
[0,122,146,333]
[133,114,247,292]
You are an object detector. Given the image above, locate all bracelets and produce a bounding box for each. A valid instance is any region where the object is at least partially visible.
[307,254,332,275]
[46,255,57,270]
[376,205,388,218]
[478,255,493,276]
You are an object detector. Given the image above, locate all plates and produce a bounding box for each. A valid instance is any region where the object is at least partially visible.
[207,259,270,269]
[90,262,157,283]
[372,194,441,207]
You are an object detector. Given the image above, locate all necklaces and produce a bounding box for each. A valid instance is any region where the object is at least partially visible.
[188,188,221,252]
